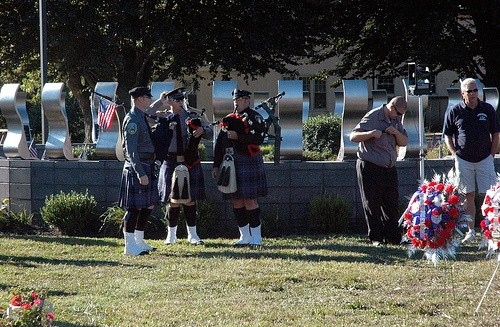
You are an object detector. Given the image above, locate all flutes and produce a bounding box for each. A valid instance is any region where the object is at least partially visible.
[179,105,220,151]
[222,91,285,155]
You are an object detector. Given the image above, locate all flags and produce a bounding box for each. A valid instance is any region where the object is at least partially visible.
[95,97,116,128]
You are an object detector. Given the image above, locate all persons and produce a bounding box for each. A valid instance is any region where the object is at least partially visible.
[119,88,167,256]
[151,86,213,245]
[349,96,408,249]
[443,77,500,242]
[210,89,267,247]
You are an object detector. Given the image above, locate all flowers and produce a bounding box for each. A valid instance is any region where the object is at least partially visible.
[480,172,500,263]
[400,168,475,268]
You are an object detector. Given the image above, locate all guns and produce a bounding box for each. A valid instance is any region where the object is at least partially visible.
[93,90,167,122]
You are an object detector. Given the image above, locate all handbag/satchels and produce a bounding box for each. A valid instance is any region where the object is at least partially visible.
[218,154,237,194]
[170,164,191,204]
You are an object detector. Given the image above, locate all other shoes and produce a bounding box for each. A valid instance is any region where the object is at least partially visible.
[461,232,476,244]
[372,240,384,247]
[488,239,498,251]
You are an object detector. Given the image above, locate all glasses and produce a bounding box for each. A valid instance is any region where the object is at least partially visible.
[463,88,479,93]
[394,106,403,116]
[140,96,152,100]
[172,96,185,102]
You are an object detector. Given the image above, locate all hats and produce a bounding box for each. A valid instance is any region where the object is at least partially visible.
[163,88,186,100]
[231,88,252,100]
[129,87,154,99]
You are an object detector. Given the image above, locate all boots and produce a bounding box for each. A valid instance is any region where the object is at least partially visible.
[164,225,178,245]
[233,223,251,246]
[185,224,204,245]
[134,230,156,250]
[123,231,148,255]
[249,224,262,246]
